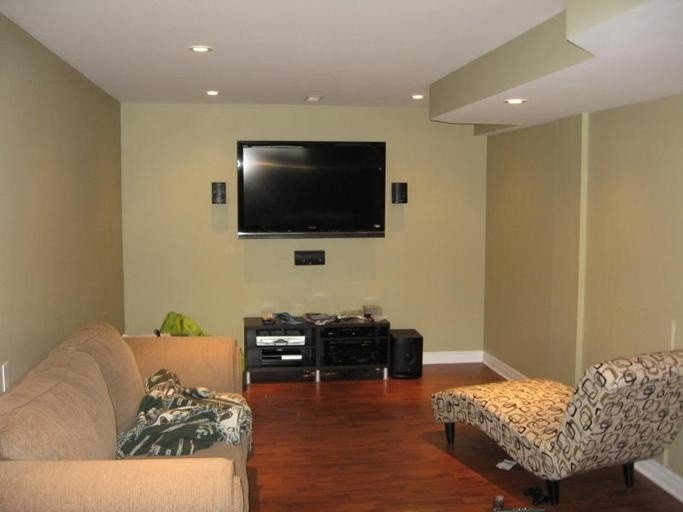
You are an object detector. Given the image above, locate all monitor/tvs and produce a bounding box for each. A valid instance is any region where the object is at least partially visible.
[236,140,387,239]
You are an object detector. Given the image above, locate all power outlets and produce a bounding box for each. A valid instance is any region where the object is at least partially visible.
[2,361,10,392]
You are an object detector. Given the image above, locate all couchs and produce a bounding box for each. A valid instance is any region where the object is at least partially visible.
[1,323,253,512]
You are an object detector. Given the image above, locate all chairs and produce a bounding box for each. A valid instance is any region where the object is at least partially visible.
[431,350,682,505]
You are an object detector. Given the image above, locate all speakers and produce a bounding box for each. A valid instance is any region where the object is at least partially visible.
[390,328,424,380]
